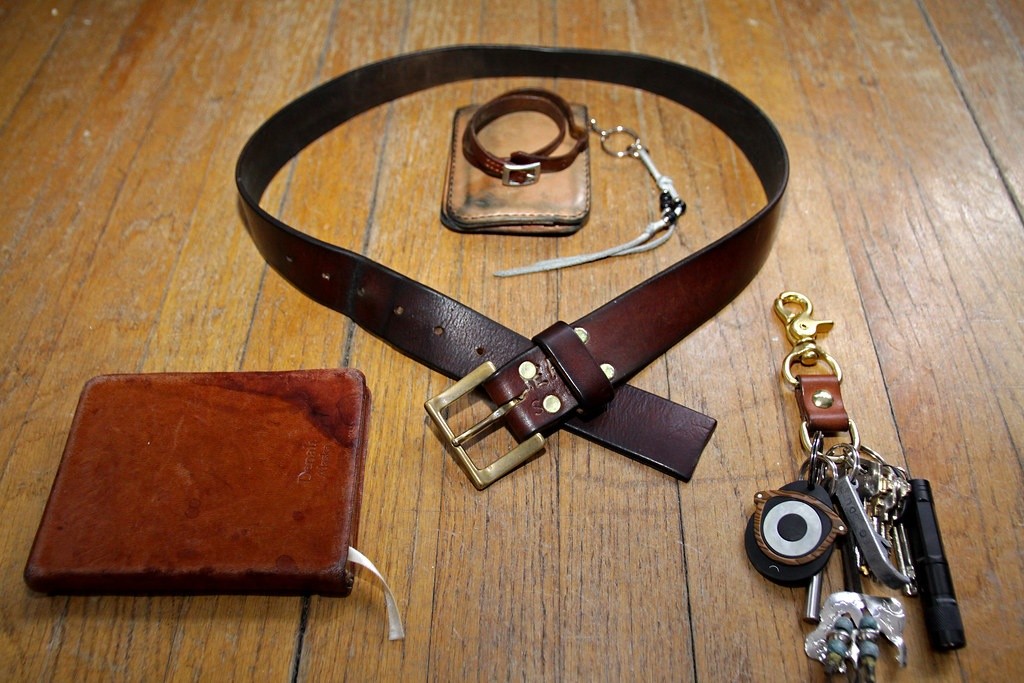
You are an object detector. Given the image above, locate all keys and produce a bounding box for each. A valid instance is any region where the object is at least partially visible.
[747,457,917,620]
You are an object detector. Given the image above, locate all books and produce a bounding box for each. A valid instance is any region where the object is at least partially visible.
[23,367,370,596]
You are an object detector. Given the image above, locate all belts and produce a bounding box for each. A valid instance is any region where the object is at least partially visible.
[234,44,787,491]
[463,87,591,187]
